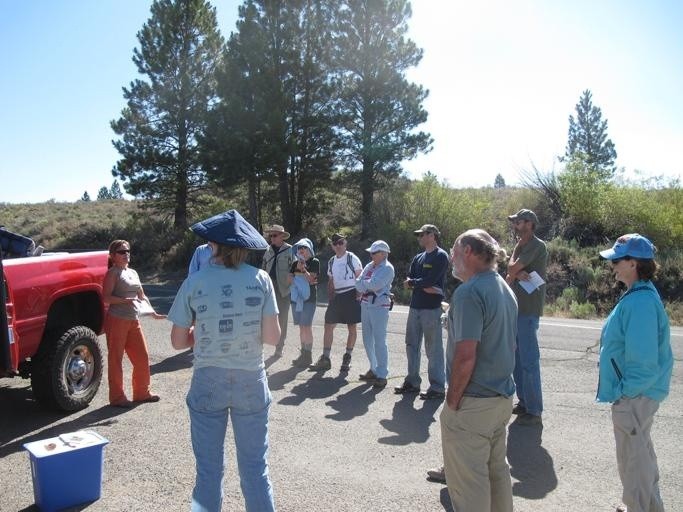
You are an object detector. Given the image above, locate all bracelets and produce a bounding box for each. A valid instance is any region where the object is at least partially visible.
[118,296,126,305]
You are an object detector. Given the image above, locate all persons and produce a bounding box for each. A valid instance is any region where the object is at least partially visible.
[393,223,449,400]
[593,232,674,512]
[427,281,463,482]
[188,241,213,352]
[354,239,395,389]
[438,227,518,512]
[494,247,510,281]
[262,224,297,358]
[308,231,364,372]
[101,239,167,409]
[504,208,547,427]
[287,237,321,367]
[166,208,283,512]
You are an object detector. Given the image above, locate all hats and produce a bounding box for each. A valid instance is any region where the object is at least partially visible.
[414,225,439,235]
[264,225,290,240]
[332,233,345,244]
[292,238,314,257]
[365,241,390,253]
[508,209,538,224]
[600,233,655,260]
[190,209,269,250]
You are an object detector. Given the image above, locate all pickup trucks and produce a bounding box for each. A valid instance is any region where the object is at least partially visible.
[0,231,108,413]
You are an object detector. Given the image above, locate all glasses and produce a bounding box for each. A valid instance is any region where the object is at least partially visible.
[297,246,307,249]
[116,250,130,254]
[512,221,521,225]
[333,242,344,245]
[269,234,277,237]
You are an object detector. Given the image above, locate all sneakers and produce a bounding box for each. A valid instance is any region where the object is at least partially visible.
[512,405,527,414]
[275,348,283,356]
[427,470,446,482]
[341,353,350,371]
[395,381,420,393]
[292,349,312,368]
[518,413,541,425]
[145,396,159,402]
[119,400,133,408]
[374,379,387,388]
[420,389,445,399]
[309,355,330,371]
[360,370,376,380]
